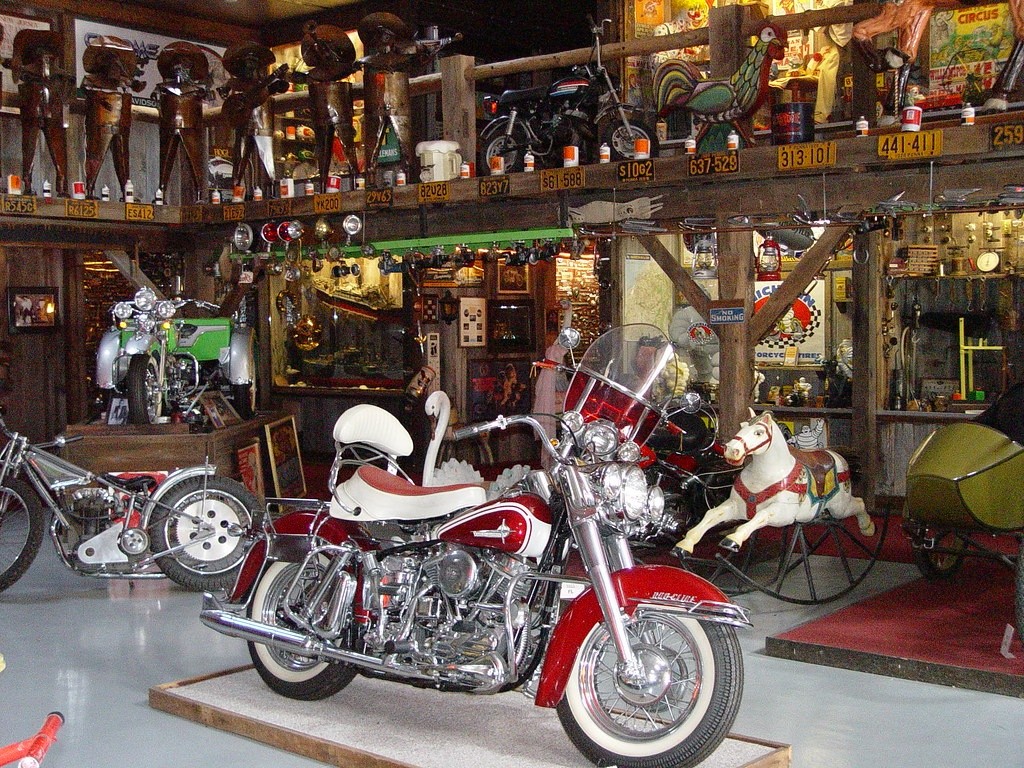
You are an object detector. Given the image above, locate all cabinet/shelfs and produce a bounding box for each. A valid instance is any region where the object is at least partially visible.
[269,108,319,182]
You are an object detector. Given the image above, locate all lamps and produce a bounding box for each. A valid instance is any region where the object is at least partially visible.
[755,231,782,282]
[690,233,719,279]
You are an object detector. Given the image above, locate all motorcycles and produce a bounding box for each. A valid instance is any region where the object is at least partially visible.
[529,327,794,545]
[193,323,753,768]
[95,276,258,426]
[0,418,263,595]
[480,15,660,176]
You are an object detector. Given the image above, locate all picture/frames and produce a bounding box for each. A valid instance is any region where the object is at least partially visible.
[493,256,531,295]
[487,298,537,353]
[203,402,227,428]
[6,287,61,334]
[196,391,243,426]
[468,358,532,422]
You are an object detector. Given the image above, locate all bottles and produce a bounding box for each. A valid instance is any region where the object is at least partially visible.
[125,180,134,203]
[524,150,534,172]
[727,130,738,150]
[600,142,610,163]
[212,189,220,204]
[856,116,868,137]
[685,135,696,154]
[305,180,314,195]
[961,103,974,125]
[254,186,262,201]
[396,169,406,186]
[460,161,469,179]
[356,174,365,190]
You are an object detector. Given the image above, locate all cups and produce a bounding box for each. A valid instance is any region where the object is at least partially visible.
[490,157,504,176]
[564,146,578,168]
[7,175,22,195]
[326,176,341,193]
[634,139,650,159]
[232,186,244,203]
[280,178,295,199]
[902,106,922,132]
[420,147,461,183]
[72,182,85,199]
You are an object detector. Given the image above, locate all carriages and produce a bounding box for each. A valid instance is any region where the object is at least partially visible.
[672,380,1024,647]
